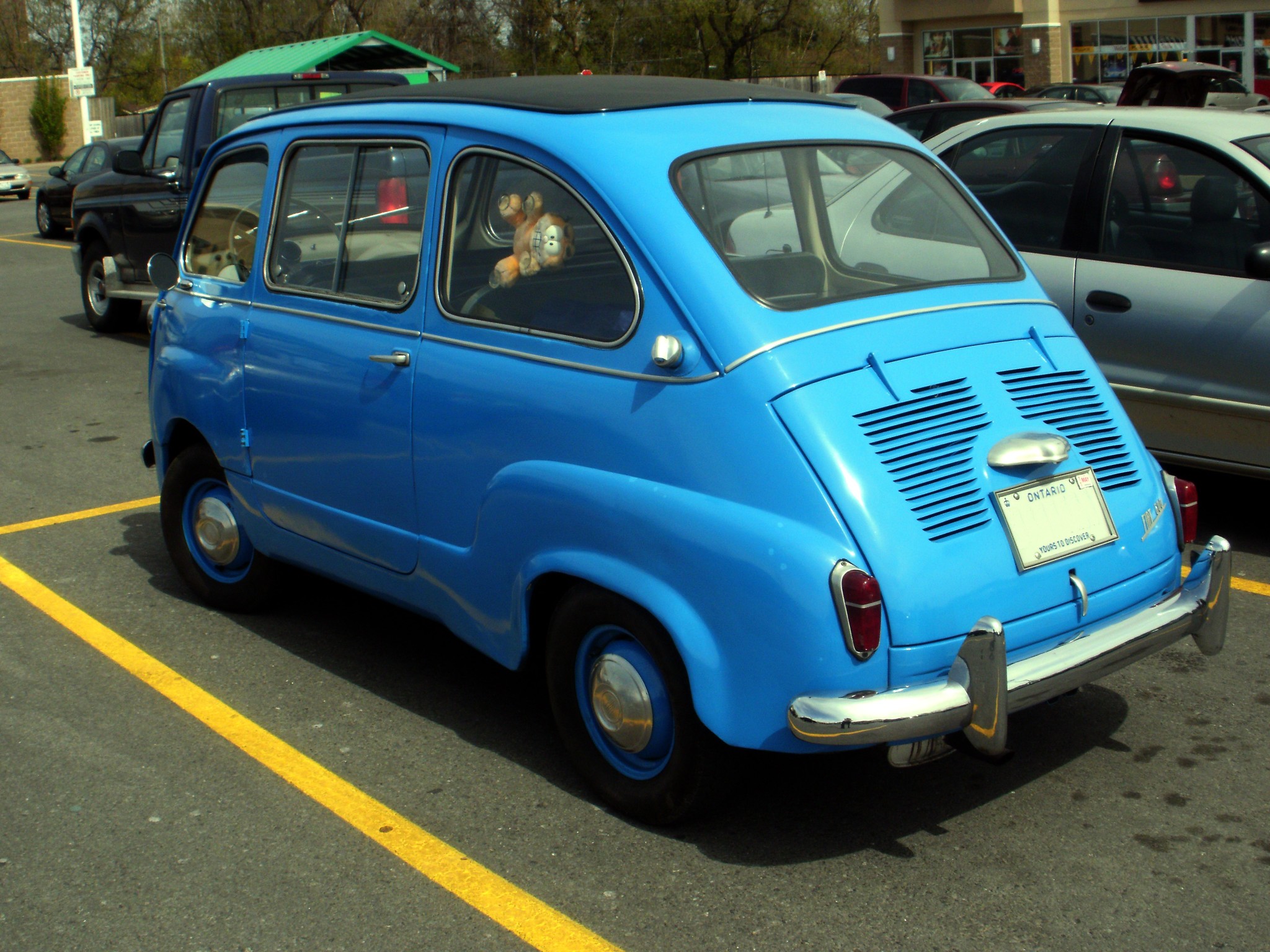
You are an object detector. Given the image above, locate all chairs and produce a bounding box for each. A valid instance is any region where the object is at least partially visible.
[1183,177,1247,255]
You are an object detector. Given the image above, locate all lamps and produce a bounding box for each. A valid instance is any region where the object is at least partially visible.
[1031,39,1041,55]
[887,47,896,61]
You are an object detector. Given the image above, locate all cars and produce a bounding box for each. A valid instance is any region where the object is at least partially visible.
[681,56,1270,235]
[0,148,32,202]
[720,100,1270,499]
[34,27,549,338]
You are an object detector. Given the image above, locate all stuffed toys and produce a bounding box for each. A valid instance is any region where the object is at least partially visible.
[488,191,577,289]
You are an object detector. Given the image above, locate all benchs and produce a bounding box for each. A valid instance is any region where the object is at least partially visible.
[462,252,825,333]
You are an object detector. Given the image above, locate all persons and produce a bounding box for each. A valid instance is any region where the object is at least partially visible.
[995,28,1023,55]
[922,30,951,57]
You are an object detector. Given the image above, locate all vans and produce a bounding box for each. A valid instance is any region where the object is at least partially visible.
[140,71,1235,813]
